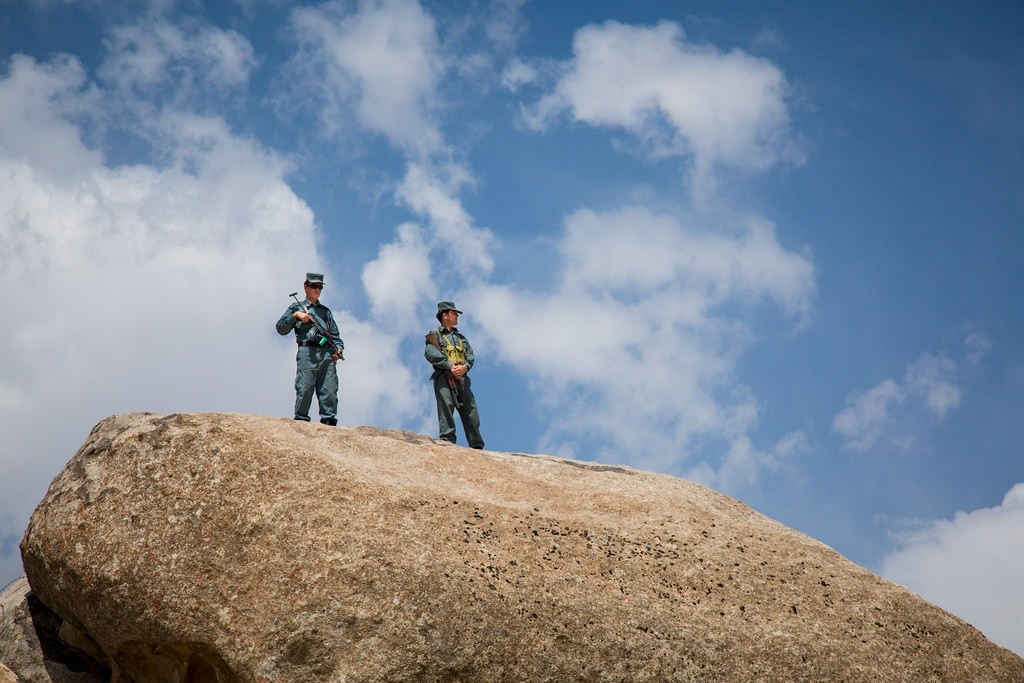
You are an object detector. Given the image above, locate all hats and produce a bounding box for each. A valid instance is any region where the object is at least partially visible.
[306,273,324,282]
[438,301,463,314]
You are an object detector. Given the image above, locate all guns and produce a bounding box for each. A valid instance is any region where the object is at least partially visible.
[425,332,463,409]
[289,292,345,361]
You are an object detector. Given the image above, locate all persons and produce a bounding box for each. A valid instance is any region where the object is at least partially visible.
[424,301,486,451]
[276,272,345,427]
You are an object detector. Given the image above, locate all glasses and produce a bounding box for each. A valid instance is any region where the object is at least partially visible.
[307,283,323,290]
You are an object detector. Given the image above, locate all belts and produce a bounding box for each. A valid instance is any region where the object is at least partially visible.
[298,341,331,347]
